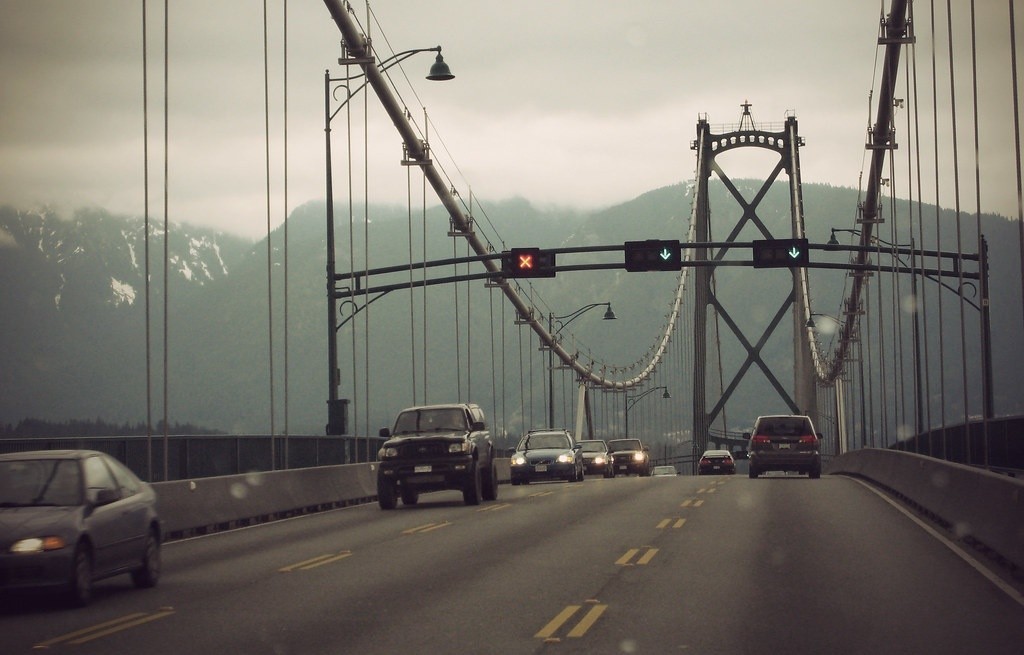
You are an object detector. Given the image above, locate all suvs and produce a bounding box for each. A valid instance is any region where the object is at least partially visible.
[376,403,498,509]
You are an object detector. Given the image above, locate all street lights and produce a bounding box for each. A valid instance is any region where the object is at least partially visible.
[625,386,672,439]
[826,227,923,434]
[805,311,867,447]
[549,301,618,431]
[323,45,455,436]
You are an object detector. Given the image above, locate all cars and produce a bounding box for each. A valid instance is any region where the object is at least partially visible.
[698,450,736,474]
[742,415,823,478]
[0,450,161,606]
[607,439,651,477]
[577,440,615,478]
[651,466,681,476]
[507,429,584,485]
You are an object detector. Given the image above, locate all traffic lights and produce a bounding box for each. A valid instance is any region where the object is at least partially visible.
[625,239,681,272]
[753,238,809,267]
[501,248,556,278]
